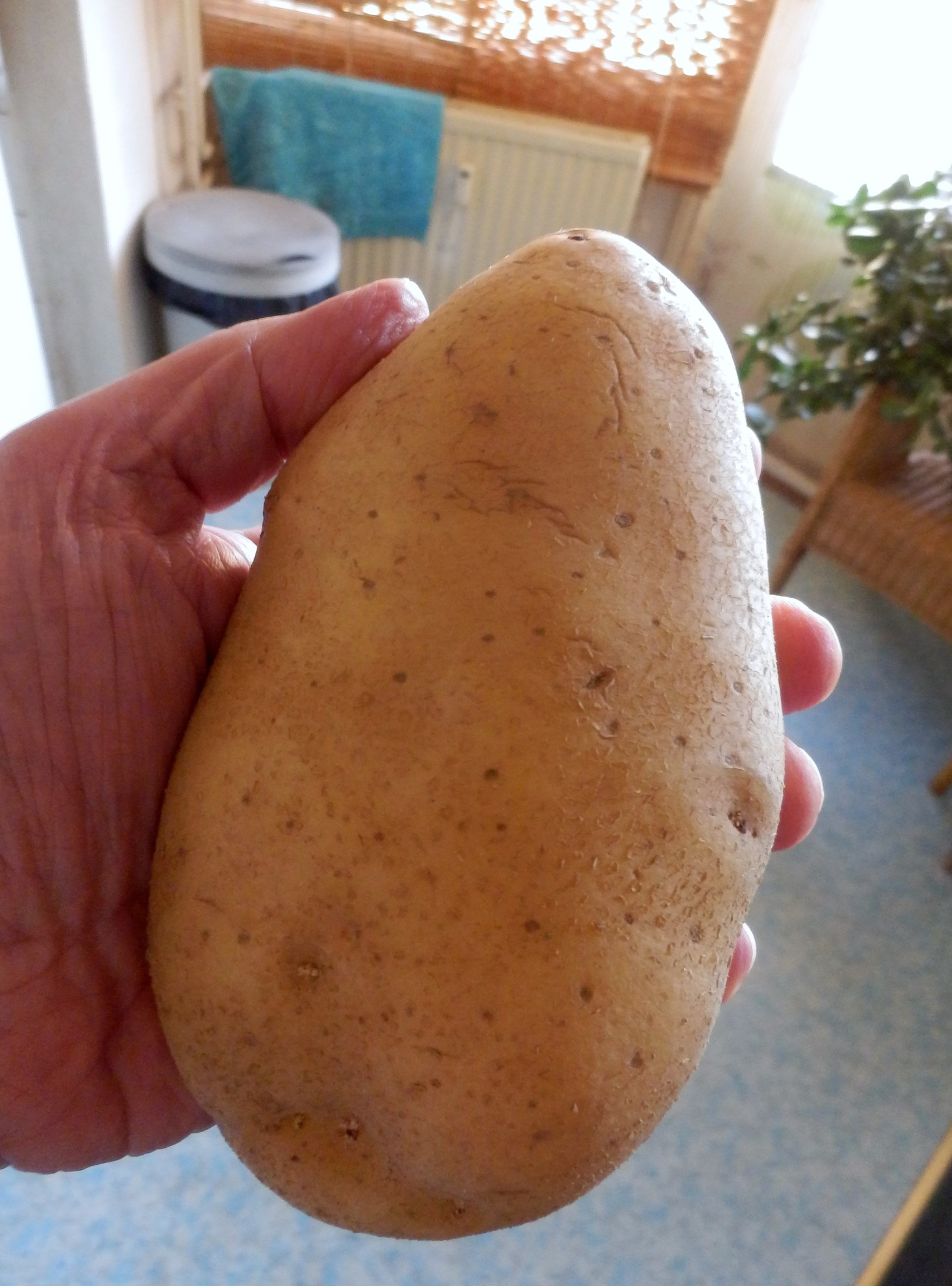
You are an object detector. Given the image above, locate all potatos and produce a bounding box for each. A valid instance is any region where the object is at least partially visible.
[146,228,788,1243]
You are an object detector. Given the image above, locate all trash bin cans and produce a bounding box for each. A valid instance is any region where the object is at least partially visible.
[141,185,341,531]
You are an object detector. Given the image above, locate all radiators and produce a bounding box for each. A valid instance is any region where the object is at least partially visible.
[322,93,656,325]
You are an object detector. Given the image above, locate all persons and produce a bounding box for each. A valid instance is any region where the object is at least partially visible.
[0,274,841,1195]
